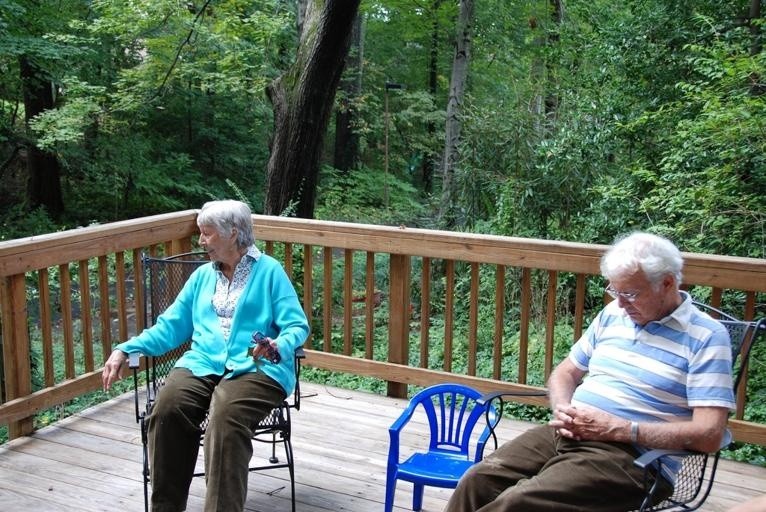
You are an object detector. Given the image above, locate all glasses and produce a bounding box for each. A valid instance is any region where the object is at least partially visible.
[250,331,281,364]
[604,281,645,300]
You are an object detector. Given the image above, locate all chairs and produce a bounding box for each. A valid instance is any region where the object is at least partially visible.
[383,382,496,512]
[128,252,305,512]
[471,302,765,512]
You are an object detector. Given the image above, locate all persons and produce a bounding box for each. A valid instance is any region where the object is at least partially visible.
[102,199,310,510]
[443,231,737,512]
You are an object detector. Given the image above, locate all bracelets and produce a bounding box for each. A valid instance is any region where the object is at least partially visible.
[632,422,639,444]
